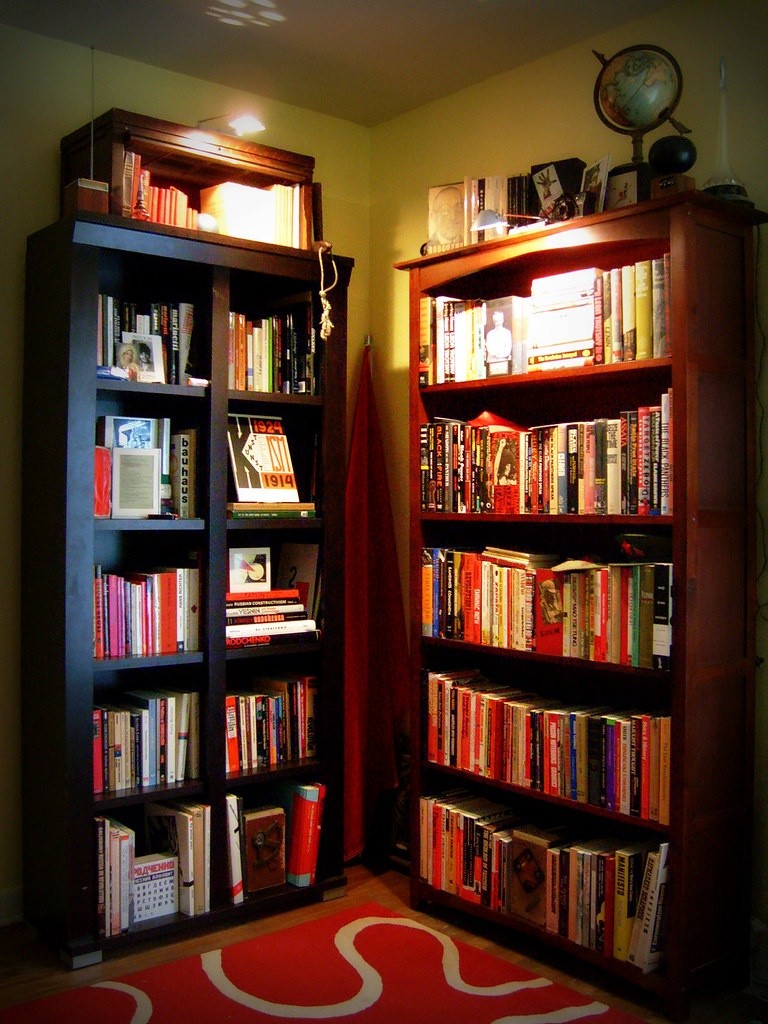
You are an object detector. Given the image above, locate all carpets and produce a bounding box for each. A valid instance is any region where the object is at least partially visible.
[0,902,650,1024]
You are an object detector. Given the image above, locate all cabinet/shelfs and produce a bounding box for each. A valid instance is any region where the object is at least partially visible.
[17,209,351,971]
[406,200,767,1024]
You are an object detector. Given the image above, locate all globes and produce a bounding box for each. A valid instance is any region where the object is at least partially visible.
[593,44,693,168]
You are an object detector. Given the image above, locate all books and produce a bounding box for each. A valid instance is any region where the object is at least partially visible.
[422,545,673,671]
[226,780,326,904]
[419,388,673,517]
[228,290,322,396]
[93,562,202,658]
[97,292,199,387]
[420,783,669,975]
[93,415,204,520]
[418,251,673,389]
[123,150,300,249]
[428,670,671,825]
[92,683,200,794]
[94,799,210,938]
[225,539,328,649]
[227,413,320,518]
[225,667,321,772]
[428,173,538,255]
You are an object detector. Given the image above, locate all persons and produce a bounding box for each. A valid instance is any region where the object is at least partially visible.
[136,343,154,372]
[428,187,463,246]
[485,310,512,376]
